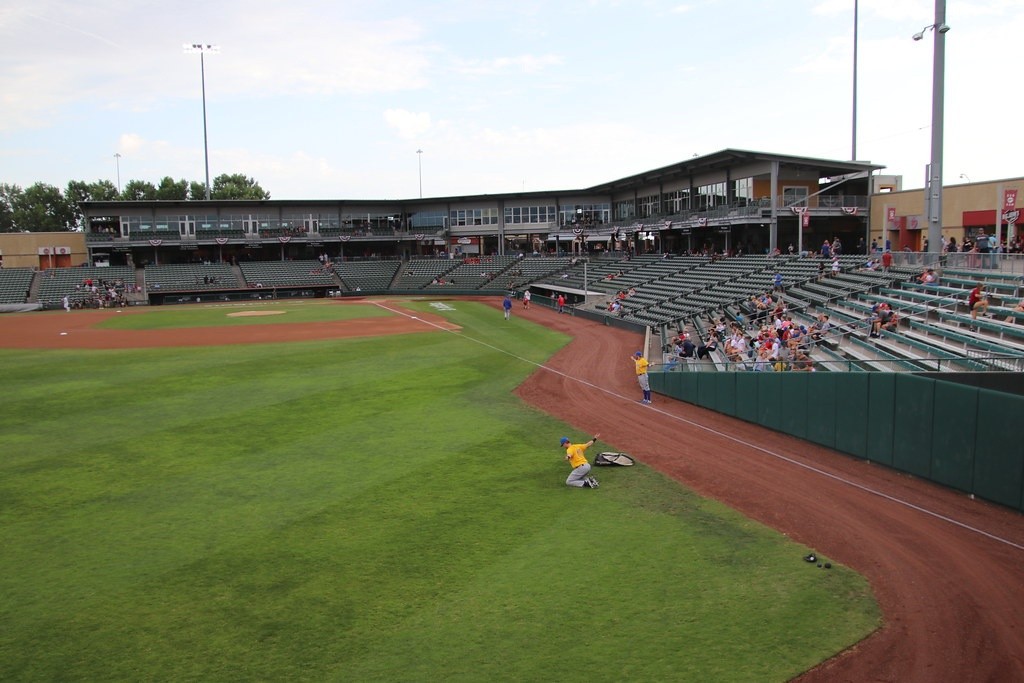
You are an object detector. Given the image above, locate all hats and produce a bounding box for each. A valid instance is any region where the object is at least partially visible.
[871,306,879,312]
[979,228,983,230]
[802,329,807,334]
[800,325,804,330]
[560,437,569,447]
[636,352,642,356]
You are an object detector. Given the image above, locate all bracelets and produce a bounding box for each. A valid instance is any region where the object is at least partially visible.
[567,456,570,460]
[592,438,597,443]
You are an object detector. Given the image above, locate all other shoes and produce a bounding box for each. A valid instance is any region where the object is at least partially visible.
[982,313,991,318]
[870,332,880,338]
[969,328,975,332]
[639,399,651,404]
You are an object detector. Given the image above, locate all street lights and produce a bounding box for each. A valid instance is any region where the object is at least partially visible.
[416,148,422,199]
[912,18,950,255]
[191,42,213,201]
[114,153,122,200]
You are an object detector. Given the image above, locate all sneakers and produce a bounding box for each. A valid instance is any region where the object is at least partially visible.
[589,476,599,487]
[584,478,593,489]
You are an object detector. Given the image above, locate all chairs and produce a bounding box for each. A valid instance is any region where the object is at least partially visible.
[144,263,239,293]
[0,266,35,305]
[330,252,643,295]
[239,260,337,289]
[36,267,135,304]
[319,226,396,238]
[85,228,305,241]
[410,226,443,234]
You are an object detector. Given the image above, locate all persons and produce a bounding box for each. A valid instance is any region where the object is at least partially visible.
[1005,300,1024,322]
[139,246,362,292]
[969,281,991,332]
[662,293,830,373]
[0,259,4,268]
[630,351,655,405]
[560,245,743,280]
[772,270,782,292]
[767,227,1024,294]
[502,268,531,320]
[870,302,900,339]
[49,276,143,313]
[605,287,634,319]
[561,209,601,232]
[261,219,401,239]
[92,221,118,242]
[550,290,578,315]
[560,432,600,489]
[504,236,556,261]
[406,246,498,285]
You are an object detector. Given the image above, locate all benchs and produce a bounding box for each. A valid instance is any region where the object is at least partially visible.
[588,253,1024,374]
